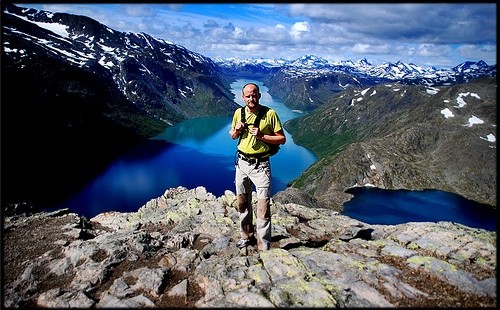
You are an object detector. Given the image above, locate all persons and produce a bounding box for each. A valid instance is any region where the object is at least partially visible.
[229,83,286,255]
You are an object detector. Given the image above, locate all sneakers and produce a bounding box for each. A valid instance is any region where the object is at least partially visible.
[236,236,255,248]
[258,242,270,251]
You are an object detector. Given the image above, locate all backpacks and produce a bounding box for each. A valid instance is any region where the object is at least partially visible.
[237,106,280,156]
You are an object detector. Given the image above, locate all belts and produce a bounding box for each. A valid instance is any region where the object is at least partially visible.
[238,153,269,164]
